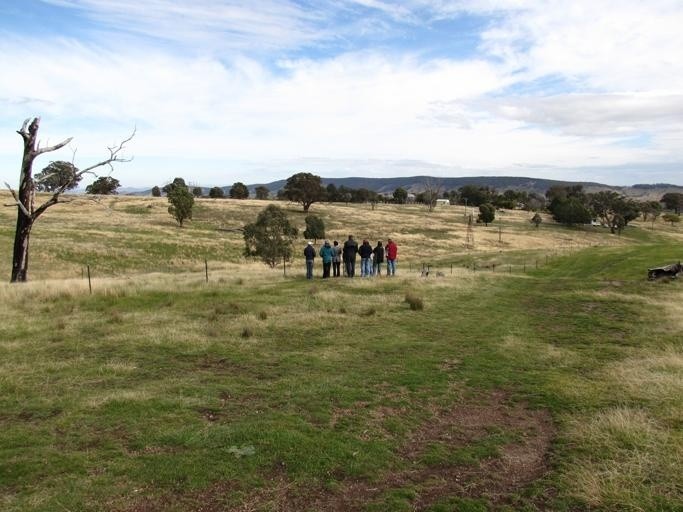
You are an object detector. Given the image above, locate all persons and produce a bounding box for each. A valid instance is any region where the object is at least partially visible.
[372,240,385,276]
[385,238,397,277]
[332,240,343,277]
[319,240,334,278]
[343,235,359,278]
[304,241,316,279]
[358,239,372,278]
